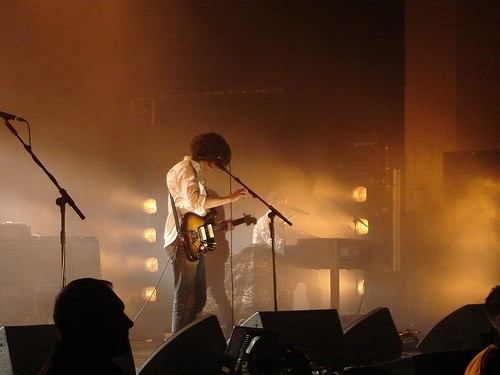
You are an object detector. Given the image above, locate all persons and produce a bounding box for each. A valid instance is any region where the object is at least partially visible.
[39,278,134,375]
[251,191,291,311]
[165,134,246,334]
[481,285,500,375]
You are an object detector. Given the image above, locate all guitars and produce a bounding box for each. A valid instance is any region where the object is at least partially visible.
[181,212,258,262]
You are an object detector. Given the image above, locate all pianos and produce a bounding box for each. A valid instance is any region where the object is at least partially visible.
[284,237,371,312]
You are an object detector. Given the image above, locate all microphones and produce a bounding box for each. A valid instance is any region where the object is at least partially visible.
[0,111,25,122]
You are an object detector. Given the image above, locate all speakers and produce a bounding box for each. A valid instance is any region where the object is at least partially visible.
[417,304,500,353]
[228,309,343,375]
[137,313,226,375]
[0,324,136,375]
[342,307,402,367]
